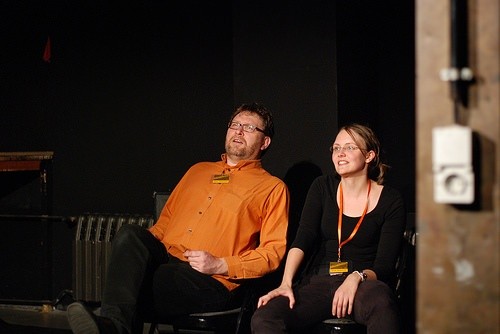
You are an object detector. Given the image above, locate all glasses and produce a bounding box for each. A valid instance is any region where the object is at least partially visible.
[330,144,370,155]
[228,121,267,135]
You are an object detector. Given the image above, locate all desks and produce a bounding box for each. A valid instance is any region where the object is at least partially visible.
[0,152,61,300]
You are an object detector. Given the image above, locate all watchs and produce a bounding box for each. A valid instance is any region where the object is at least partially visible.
[354,270,367,282]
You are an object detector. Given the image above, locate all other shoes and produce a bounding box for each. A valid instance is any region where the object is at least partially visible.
[67,302,120,333]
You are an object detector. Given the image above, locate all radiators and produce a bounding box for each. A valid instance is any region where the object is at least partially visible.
[74,212,155,303]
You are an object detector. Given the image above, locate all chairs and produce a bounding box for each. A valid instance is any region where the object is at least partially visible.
[148,191,256,333]
[318,225,415,333]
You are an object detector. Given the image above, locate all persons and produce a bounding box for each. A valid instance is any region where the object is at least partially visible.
[66,103,290,334]
[251,120,406,334]
